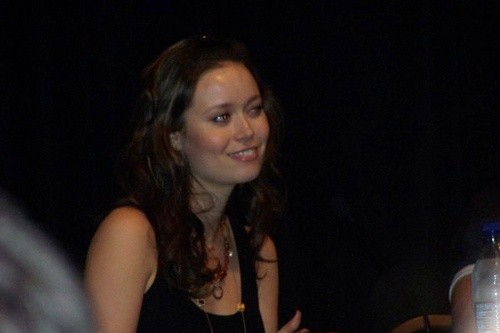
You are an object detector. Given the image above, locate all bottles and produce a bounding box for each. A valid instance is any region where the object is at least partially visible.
[472,224,500,333]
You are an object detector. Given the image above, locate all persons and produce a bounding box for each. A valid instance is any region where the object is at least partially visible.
[83,32,312,333]
[450,260,479,332]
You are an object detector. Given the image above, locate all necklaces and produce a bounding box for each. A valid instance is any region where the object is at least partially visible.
[190,212,250,332]
